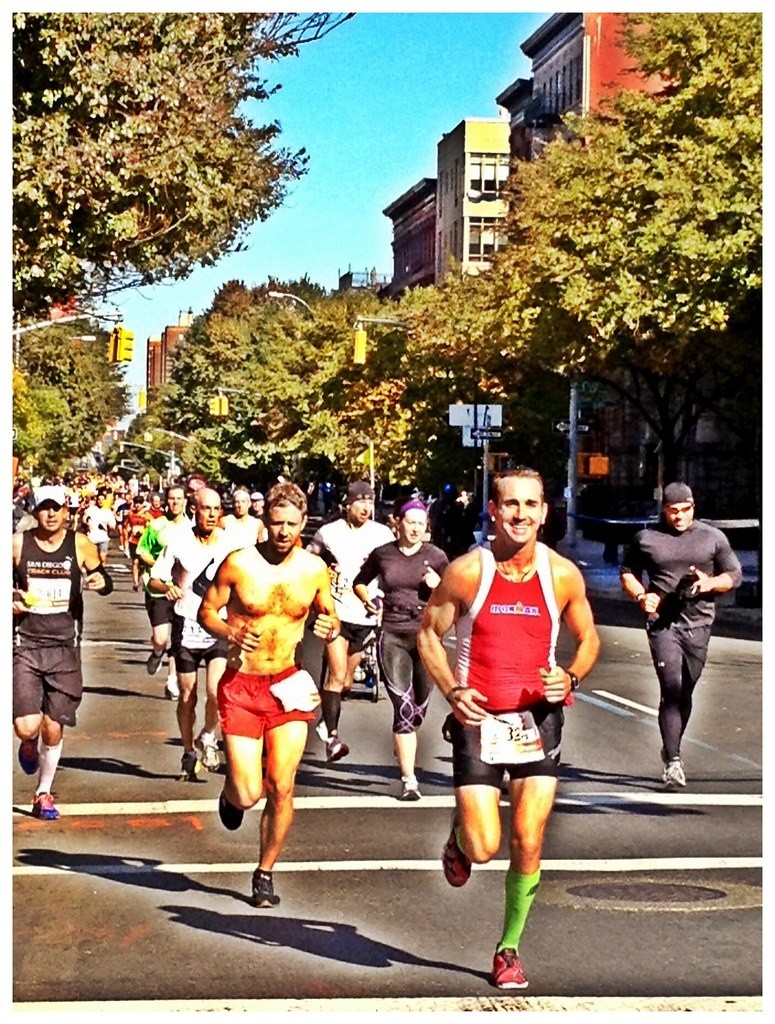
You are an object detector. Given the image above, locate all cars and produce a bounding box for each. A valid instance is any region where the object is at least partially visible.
[556,517,762,612]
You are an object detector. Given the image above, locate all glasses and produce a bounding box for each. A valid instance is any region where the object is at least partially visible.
[667,502,693,514]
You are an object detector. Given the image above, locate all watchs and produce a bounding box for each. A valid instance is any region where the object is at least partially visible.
[565,671,580,691]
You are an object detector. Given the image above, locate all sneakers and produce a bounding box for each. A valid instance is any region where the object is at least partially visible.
[400,776,421,801]
[251,868,281,909]
[31,791,62,820]
[17,735,41,776]
[325,736,350,764]
[179,750,201,782]
[146,650,164,674]
[195,729,220,774]
[164,676,180,701]
[441,807,472,887]
[315,720,331,743]
[219,791,245,830]
[660,747,687,789]
[492,943,529,989]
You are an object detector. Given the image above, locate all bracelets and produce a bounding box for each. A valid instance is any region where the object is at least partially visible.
[446,686,462,702]
[328,627,338,642]
[633,593,643,602]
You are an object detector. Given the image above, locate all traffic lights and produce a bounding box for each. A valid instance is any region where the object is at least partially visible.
[207,396,221,417]
[114,326,135,361]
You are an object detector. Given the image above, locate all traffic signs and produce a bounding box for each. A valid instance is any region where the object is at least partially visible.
[555,419,593,438]
[470,428,502,440]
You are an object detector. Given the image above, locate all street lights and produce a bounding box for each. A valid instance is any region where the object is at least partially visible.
[117,439,176,480]
[143,427,193,477]
[269,291,377,518]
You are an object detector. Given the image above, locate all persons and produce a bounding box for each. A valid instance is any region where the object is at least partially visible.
[197,482,341,907]
[219,486,264,560]
[136,485,197,700]
[12,486,116,820]
[619,482,743,791]
[427,481,476,559]
[147,487,240,782]
[13,466,224,589]
[411,468,600,990]
[305,480,397,763]
[352,502,452,802]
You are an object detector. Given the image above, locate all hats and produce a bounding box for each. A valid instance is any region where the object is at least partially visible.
[399,498,427,518]
[251,491,263,501]
[344,482,373,504]
[33,486,66,507]
[664,483,694,507]
